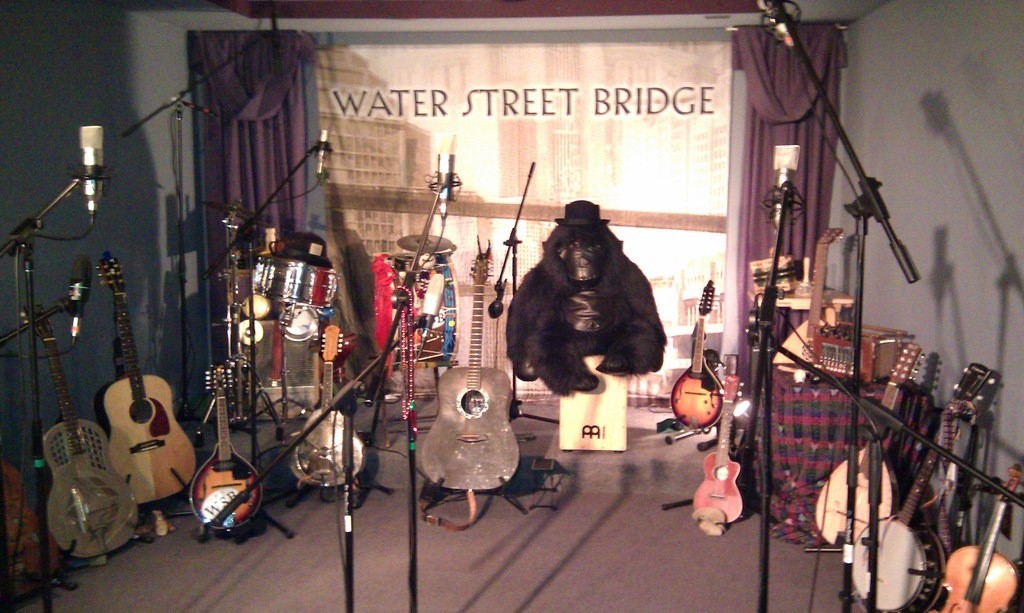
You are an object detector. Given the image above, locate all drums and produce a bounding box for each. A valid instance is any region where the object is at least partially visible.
[255,254,339,310]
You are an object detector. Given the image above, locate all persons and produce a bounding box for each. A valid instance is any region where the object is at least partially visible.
[271,231,357,502]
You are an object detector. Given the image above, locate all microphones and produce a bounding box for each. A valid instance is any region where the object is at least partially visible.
[758,0,797,51]
[183,101,217,117]
[421,274,444,341]
[748,297,760,346]
[488,279,508,319]
[270,0,281,46]
[80,126,105,217]
[773,144,801,229]
[70,281,85,342]
[317,129,329,179]
[438,154,456,218]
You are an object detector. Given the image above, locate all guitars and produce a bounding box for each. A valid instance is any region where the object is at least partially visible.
[1,462,60,600]
[851,362,997,613]
[419,252,522,493]
[18,302,141,559]
[693,375,745,525]
[669,280,726,431]
[94,249,197,507]
[289,323,366,488]
[189,362,265,532]
[772,225,849,380]
[936,462,1024,613]
[813,341,926,551]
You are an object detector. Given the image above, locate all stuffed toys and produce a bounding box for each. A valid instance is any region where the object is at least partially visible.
[233,221,267,269]
[415,252,437,316]
[506,200,667,397]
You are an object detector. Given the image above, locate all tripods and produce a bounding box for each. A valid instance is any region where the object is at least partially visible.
[192,211,314,449]
[498,162,563,425]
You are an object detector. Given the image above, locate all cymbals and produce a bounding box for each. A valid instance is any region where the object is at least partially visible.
[396,234,453,253]
